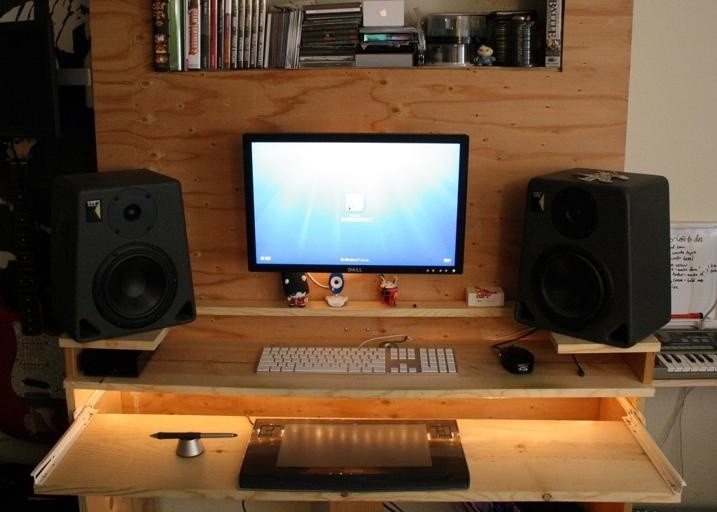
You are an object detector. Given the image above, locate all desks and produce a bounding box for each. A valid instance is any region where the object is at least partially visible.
[25,299,693,512]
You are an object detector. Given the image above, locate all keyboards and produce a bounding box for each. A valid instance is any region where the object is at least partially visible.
[254,346,460,376]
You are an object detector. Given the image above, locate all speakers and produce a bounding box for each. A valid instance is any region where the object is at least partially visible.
[49,169,197,344]
[514,167,671,349]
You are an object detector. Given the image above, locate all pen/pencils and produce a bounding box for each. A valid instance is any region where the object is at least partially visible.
[150,433,237,439]
[671,313,704,319]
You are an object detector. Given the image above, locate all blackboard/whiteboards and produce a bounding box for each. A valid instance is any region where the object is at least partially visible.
[659,222,717,330]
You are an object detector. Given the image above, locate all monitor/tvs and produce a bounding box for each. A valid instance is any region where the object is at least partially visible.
[243,132,470,275]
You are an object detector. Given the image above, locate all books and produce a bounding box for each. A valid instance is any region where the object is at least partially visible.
[151,1,270,72]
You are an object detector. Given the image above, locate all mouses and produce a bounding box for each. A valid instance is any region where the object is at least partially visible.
[499,346,534,374]
